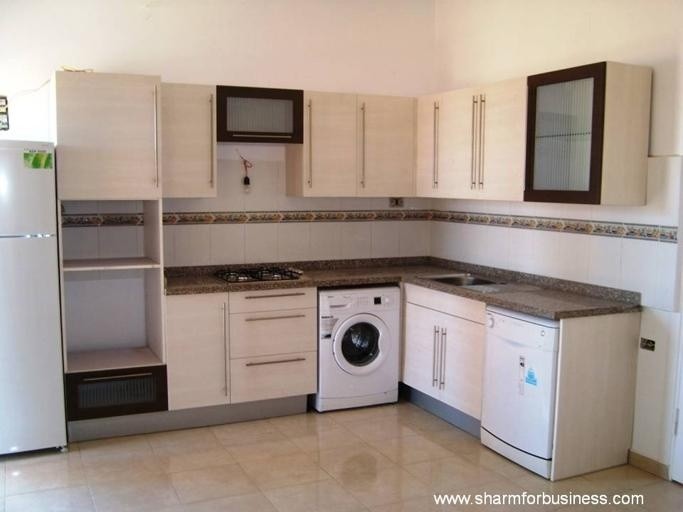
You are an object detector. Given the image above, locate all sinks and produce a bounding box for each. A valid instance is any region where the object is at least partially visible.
[424,271,496,288]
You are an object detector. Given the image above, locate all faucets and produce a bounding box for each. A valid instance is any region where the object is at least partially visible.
[494,273,510,285]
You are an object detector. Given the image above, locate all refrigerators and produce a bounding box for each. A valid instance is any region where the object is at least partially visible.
[0,139,67,457]
[472,302,560,482]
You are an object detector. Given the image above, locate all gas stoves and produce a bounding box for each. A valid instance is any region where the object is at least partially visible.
[210,267,300,283]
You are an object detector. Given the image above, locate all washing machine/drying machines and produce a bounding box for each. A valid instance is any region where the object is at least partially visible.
[312,286,404,412]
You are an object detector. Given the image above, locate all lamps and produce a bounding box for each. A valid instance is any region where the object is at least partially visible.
[240,155,254,195]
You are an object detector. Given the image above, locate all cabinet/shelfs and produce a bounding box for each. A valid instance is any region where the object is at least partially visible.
[54,70,218,201]
[398,280,485,441]
[450,75,527,203]
[228,285,320,424]
[215,83,305,144]
[286,90,450,199]
[165,292,232,430]
[527,60,653,208]
[57,203,169,442]
